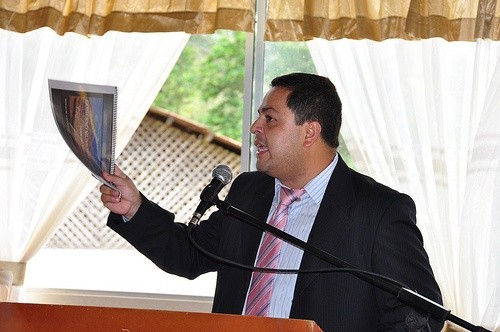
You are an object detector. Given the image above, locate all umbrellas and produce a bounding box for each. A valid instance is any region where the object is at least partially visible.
[0,0,500,181]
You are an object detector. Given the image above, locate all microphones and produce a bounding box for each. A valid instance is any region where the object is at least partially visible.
[188,164,233,231]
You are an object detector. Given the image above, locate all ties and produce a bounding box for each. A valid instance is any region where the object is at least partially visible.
[245,186,307,316]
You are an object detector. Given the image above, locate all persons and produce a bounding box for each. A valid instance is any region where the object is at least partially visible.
[99,70,445,332]
